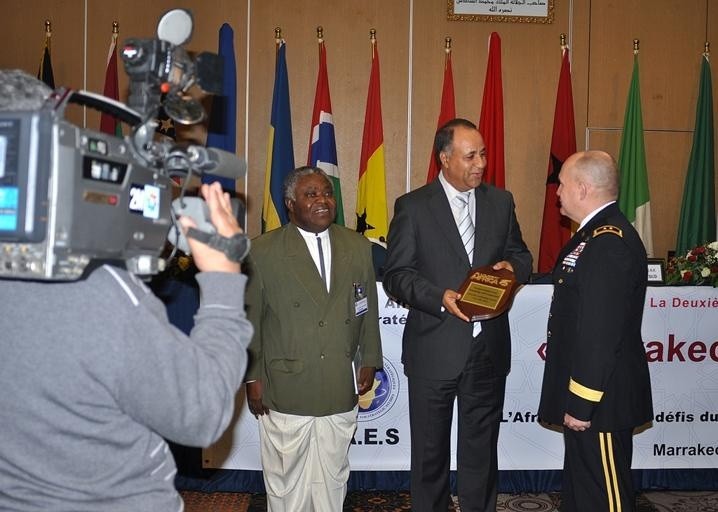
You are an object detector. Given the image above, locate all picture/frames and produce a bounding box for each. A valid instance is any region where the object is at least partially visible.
[446,0,555,23]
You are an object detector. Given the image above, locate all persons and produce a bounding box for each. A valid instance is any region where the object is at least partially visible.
[1,66,256,511]
[382,118,534,510]
[535,150,654,512]
[241,162,384,510]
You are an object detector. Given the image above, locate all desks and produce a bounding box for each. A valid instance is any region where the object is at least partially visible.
[140,275,718,494]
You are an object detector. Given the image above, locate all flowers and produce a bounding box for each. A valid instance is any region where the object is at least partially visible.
[665,238,718,289]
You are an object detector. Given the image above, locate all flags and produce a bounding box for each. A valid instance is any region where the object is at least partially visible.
[617,50,655,258]
[37,46,55,90]
[676,53,718,257]
[356,42,389,282]
[100,37,123,139]
[261,41,298,232]
[306,43,346,226]
[426,50,456,187]
[478,32,505,189]
[538,47,577,274]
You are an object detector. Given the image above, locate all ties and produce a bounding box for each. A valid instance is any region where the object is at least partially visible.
[456,192,482,338]
